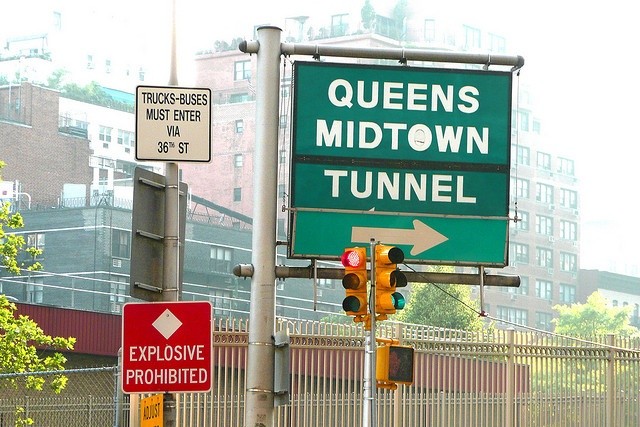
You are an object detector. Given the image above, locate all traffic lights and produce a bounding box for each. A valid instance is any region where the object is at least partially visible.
[341,248,367,316]
[377,344,415,386]
[375,246,407,313]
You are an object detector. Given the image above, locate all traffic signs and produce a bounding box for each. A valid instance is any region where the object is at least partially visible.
[136,86,211,162]
[287,61,511,267]
[121,301,213,394]
